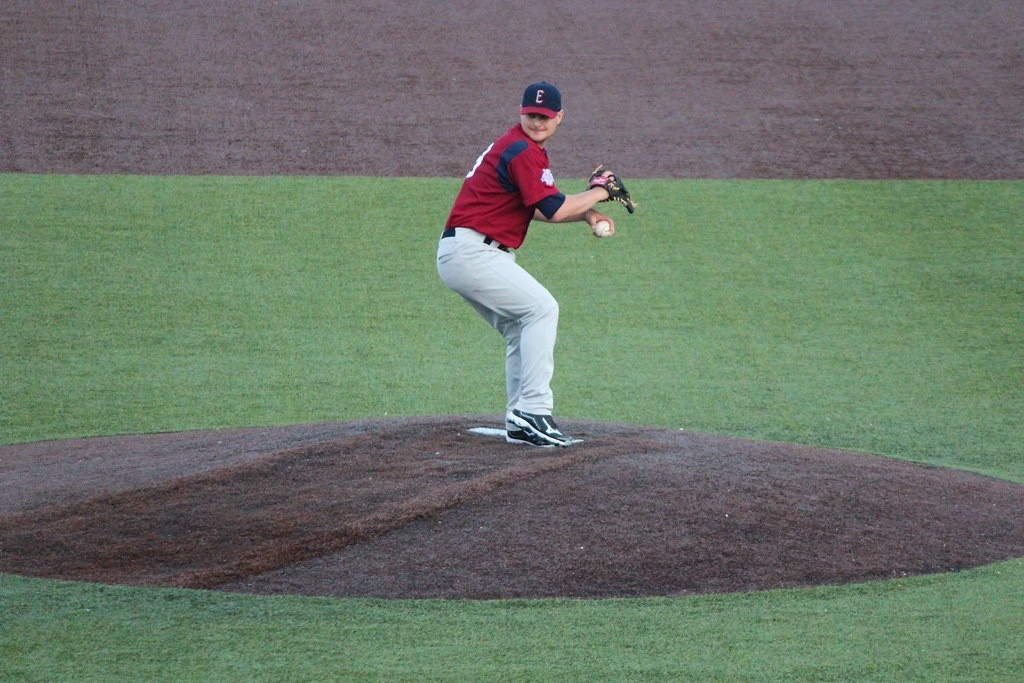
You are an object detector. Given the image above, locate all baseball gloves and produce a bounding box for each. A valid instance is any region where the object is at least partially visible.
[585,170,634,215]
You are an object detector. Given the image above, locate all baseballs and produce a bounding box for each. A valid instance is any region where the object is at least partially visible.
[595,221,610,238]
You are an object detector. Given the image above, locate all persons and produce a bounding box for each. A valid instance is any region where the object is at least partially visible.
[437,81,635,447]
